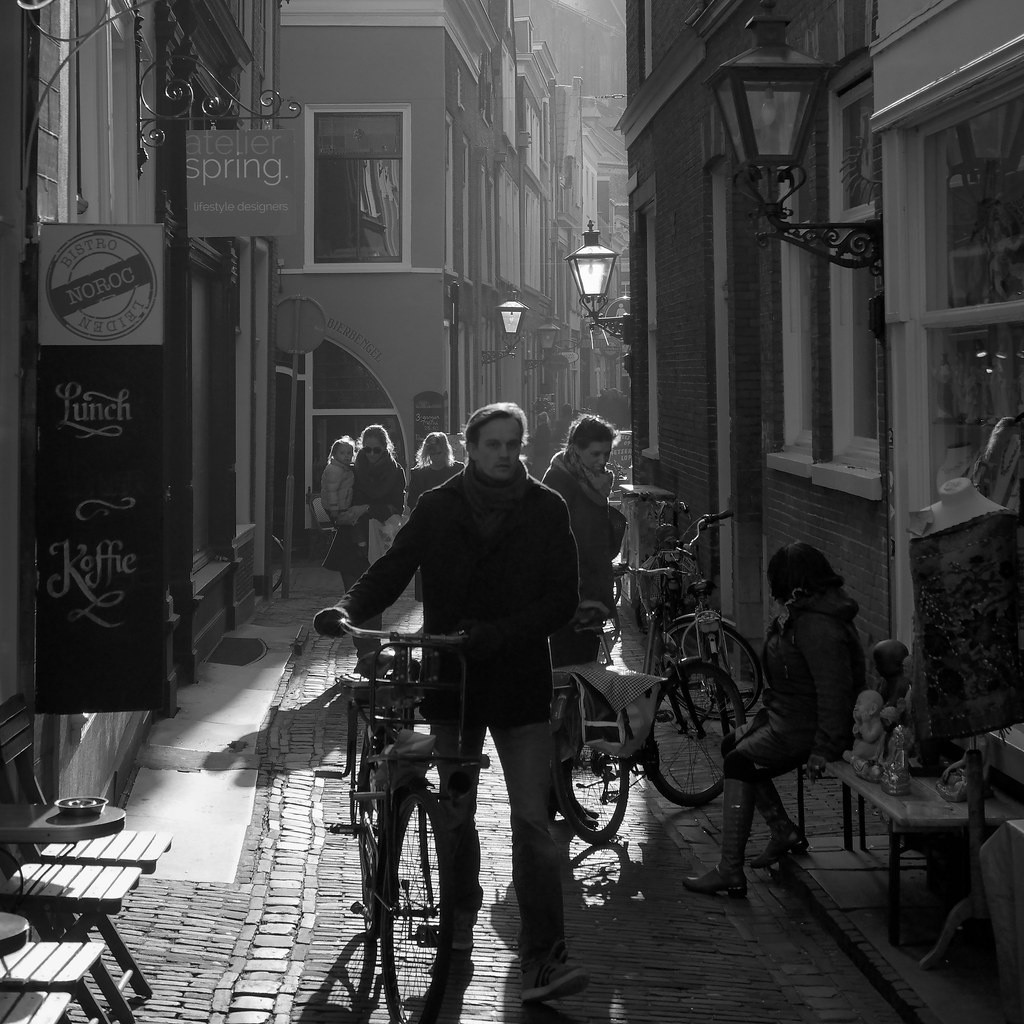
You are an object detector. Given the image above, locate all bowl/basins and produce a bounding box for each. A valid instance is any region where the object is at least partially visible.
[54,796,109,814]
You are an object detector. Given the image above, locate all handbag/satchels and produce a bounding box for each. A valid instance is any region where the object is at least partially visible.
[320,529,346,571]
[367,504,410,565]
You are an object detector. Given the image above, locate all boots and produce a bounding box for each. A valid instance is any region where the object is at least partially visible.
[751,776,809,869]
[682,779,754,900]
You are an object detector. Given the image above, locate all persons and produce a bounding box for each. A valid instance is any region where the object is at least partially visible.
[682,543,865,898]
[535,412,549,449]
[881,725,912,784]
[940,734,988,793]
[541,418,626,830]
[896,656,914,723]
[321,438,367,547]
[910,477,1024,970]
[842,690,884,765]
[323,425,407,674]
[315,403,587,1000]
[406,431,464,602]
[873,640,909,706]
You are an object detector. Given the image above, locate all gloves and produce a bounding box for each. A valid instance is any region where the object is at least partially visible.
[312,607,352,637]
[454,621,502,660]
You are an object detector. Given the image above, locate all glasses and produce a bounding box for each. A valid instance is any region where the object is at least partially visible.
[364,446,382,455]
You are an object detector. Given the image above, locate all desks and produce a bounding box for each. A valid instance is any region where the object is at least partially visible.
[0,912,31,958]
[0,799,128,844]
[795,756,1024,949]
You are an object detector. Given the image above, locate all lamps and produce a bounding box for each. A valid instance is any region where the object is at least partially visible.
[702,2,887,272]
[563,220,640,345]
[523,316,560,369]
[481,291,528,365]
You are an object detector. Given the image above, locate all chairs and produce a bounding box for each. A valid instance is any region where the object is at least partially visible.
[304,494,335,562]
[0,695,172,1024]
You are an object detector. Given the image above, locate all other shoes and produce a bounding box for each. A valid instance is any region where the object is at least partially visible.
[548,790,599,827]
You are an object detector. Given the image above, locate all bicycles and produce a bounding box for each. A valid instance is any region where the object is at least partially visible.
[549,507,763,847]
[328,615,491,1024]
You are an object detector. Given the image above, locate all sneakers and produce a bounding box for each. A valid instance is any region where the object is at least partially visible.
[451,913,478,950]
[520,940,588,1002]
